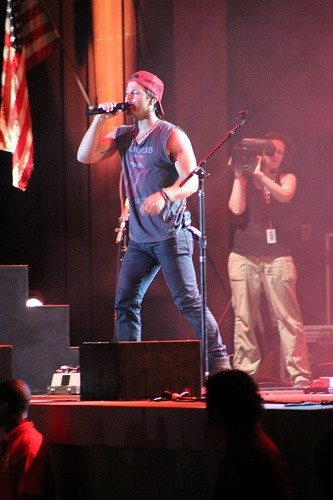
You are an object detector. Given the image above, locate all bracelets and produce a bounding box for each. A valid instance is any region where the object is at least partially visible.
[158,187,172,204]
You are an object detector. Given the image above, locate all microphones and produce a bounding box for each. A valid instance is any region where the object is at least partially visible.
[84,103,129,115]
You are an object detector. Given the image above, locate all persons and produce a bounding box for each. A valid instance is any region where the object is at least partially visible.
[227,130,312,389]
[202,369,291,500]
[75,70,233,380]
[0,377,50,500]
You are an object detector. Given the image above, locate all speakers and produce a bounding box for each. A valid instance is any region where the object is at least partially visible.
[78,338,202,400]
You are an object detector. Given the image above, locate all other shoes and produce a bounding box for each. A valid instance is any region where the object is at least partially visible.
[294,374,313,389]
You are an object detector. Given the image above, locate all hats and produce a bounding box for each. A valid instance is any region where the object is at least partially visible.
[127,70,166,119]
